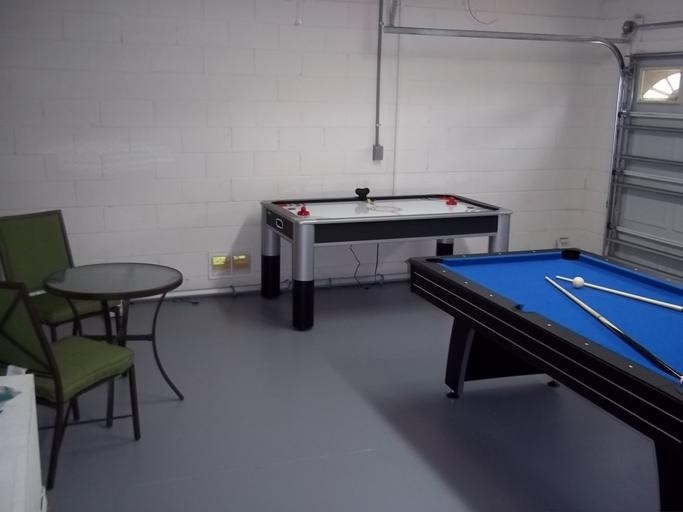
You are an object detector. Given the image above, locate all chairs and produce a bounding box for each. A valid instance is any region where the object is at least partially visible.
[3,209,122,340]
[1,281,144,495]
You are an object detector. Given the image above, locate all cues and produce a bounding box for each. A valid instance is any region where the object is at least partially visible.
[554,274,683,314]
[544,274,683,381]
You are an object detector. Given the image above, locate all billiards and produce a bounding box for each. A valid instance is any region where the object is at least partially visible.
[571,276,585,290]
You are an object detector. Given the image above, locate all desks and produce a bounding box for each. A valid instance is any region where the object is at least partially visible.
[404,246,682,512]
[44,256,185,441]
